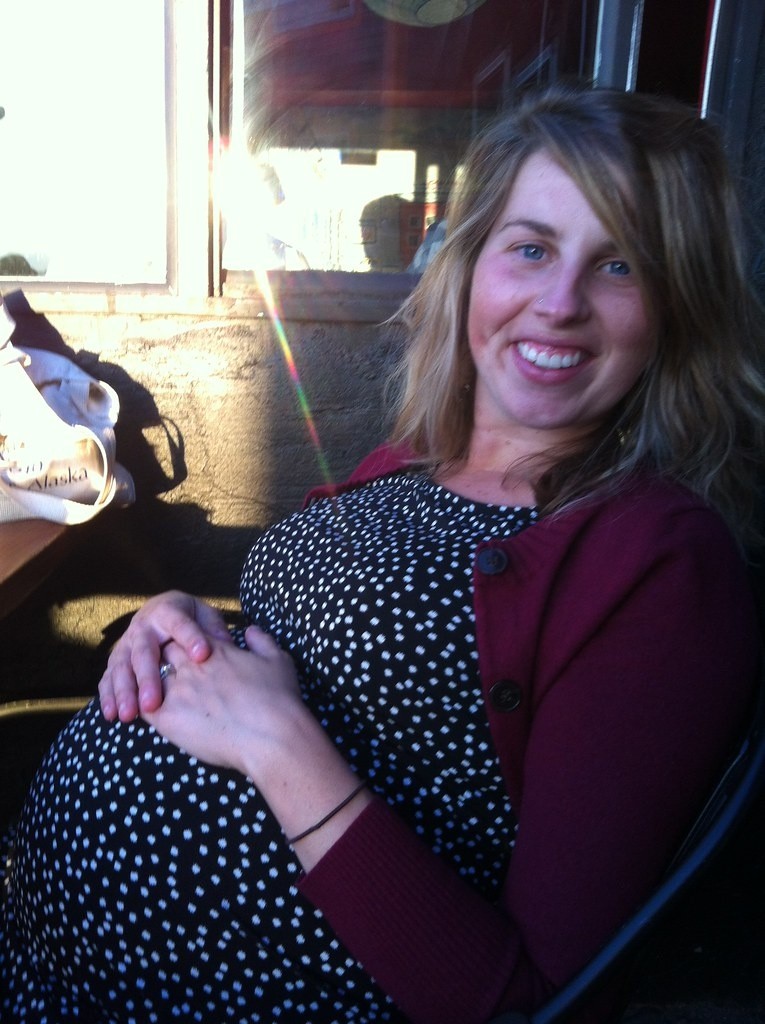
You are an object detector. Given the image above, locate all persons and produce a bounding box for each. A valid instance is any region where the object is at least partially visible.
[2,81,759,1024]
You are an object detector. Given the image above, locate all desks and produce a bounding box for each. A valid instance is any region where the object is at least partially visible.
[0,514,110,621]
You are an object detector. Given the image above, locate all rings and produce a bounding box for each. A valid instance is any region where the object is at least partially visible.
[157,662,175,679]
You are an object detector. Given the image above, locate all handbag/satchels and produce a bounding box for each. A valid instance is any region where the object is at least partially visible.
[0,288,137,524]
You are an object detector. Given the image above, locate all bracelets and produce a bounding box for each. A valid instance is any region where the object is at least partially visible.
[288,779,370,844]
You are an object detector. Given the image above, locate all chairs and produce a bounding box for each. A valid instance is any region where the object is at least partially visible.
[0,697,764,1024]
